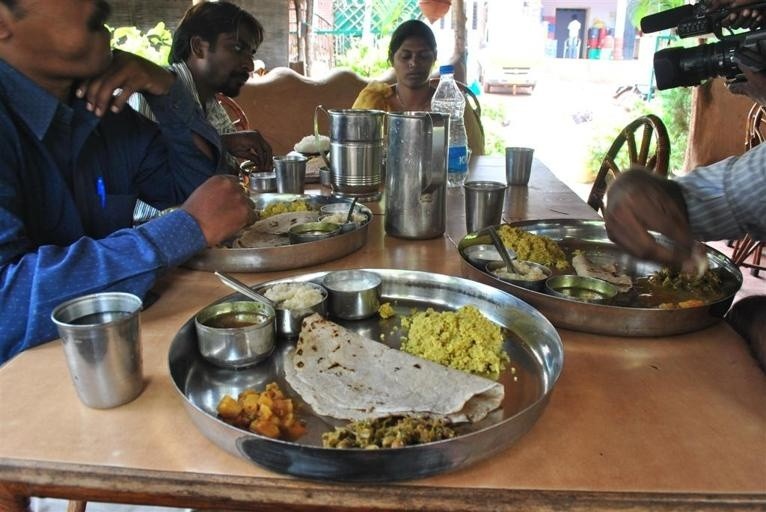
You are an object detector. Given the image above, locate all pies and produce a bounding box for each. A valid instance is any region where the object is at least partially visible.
[229,209,321,250]
[281,311,506,428]
[571,254,633,295]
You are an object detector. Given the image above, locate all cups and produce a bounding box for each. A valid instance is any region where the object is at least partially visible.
[271,154,308,194]
[462,179,508,234]
[505,146,534,185]
[49,289,145,414]
[319,166,334,200]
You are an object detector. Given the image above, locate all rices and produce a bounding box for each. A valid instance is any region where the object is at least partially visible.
[272,134,332,178]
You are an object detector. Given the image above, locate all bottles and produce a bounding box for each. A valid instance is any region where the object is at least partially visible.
[430,64,469,187]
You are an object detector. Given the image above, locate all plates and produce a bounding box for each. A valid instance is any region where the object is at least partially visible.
[302,173,321,186]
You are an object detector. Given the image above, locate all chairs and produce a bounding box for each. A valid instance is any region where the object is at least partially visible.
[585,113,671,221]
[562,37,582,59]
[215,93,251,184]
[725,98,766,277]
[428,76,481,121]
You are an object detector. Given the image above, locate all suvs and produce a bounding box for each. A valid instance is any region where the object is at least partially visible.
[475,40,542,96]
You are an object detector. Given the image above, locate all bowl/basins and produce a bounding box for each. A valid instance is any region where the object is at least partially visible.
[462,243,515,267]
[190,301,277,370]
[249,172,276,192]
[541,273,619,308]
[316,214,366,232]
[485,259,553,292]
[320,267,384,322]
[252,283,328,339]
[318,201,362,213]
[287,221,341,246]
[292,142,330,158]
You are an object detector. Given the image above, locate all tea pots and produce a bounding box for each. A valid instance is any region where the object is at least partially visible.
[311,104,387,200]
[382,110,451,241]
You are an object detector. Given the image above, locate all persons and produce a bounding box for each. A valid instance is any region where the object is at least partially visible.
[565,14,581,60]
[604,139,764,369]
[726,63,764,113]
[349,20,486,158]
[0,0,255,361]
[114,0,273,224]
